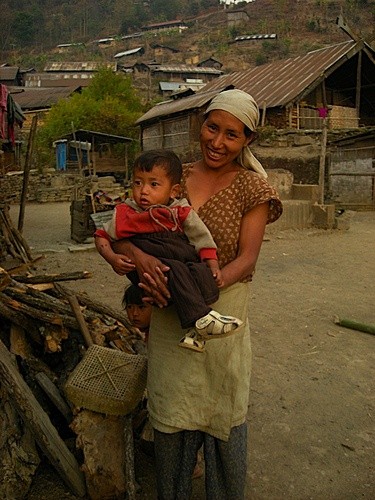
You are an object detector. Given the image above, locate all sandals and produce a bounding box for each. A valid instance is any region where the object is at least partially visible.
[178,327,206,352]
[195,310,245,341]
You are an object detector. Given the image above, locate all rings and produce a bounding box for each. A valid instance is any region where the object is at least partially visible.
[153,291,157,294]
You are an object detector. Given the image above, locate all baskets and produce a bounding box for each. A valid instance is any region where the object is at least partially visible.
[64,343,148,414]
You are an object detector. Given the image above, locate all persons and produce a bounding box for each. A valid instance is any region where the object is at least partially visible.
[114,89,284,500]
[122,284,207,479]
[93,151,246,354]
